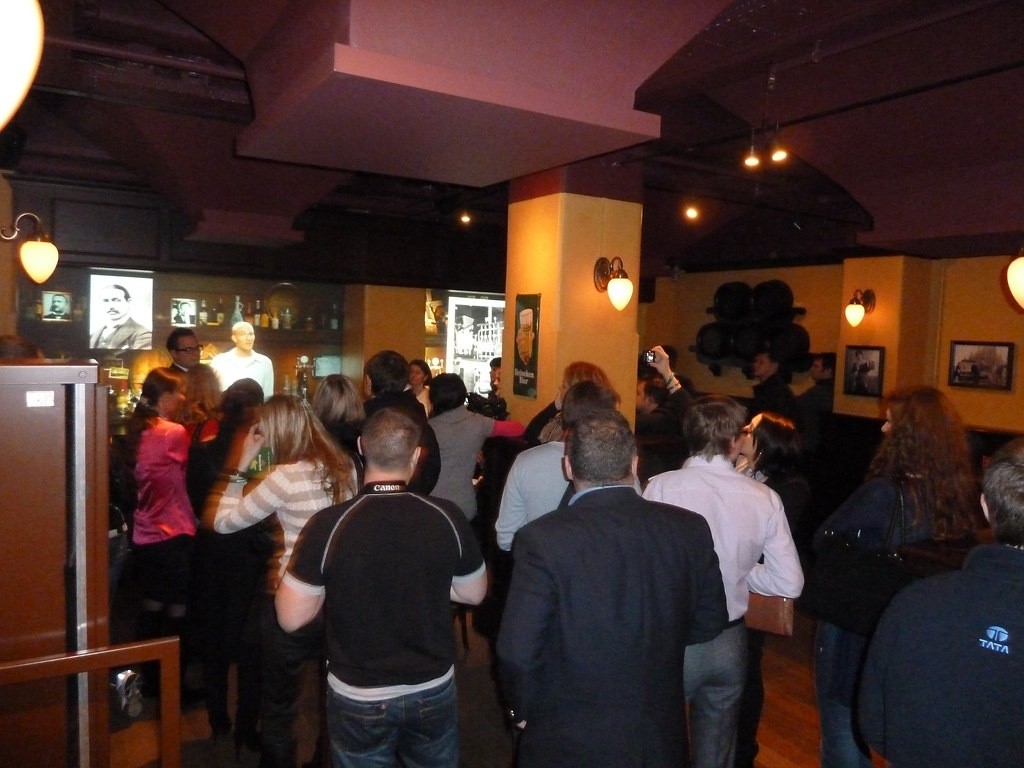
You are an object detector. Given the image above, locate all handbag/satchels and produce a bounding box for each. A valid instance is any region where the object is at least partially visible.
[806,485,913,641]
[745,590,793,636]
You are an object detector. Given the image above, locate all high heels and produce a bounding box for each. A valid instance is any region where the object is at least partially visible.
[232,725,260,758]
[207,713,232,742]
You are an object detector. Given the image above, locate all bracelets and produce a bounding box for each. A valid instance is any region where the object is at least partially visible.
[230,468,248,478]
[668,382,679,391]
[665,372,674,387]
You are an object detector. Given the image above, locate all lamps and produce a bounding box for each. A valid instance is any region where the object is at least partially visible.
[1004,251,1024,315]
[0,212,62,286]
[767,116,793,165]
[593,255,634,315]
[742,118,761,170]
[842,286,880,327]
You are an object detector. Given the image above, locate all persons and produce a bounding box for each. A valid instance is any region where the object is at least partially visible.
[850,350,875,392]
[173,302,195,324]
[802,386,972,768]
[800,358,833,422]
[274,409,488,768]
[497,410,729,767]
[495,362,641,551]
[640,395,805,768]
[118,328,524,768]
[749,350,797,422]
[955,359,980,385]
[858,453,1024,768]
[636,344,696,490]
[90,285,152,349]
[648,344,807,535]
[211,322,277,402]
[44,295,70,320]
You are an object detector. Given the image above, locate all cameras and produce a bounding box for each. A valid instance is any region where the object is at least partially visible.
[643,351,655,363]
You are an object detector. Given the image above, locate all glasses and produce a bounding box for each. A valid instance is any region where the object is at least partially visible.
[176,345,204,356]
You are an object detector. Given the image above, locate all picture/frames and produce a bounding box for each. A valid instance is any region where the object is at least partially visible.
[945,341,1014,392]
[167,296,197,327]
[842,344,887,400]
[513,292,542,399]
[83,265,159,355]
[36,290,75,325]
